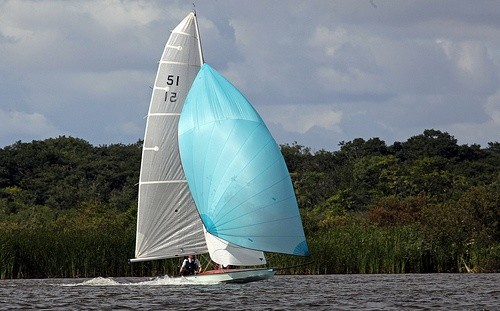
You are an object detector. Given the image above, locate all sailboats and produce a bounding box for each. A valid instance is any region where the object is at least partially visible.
[127,3,315,285]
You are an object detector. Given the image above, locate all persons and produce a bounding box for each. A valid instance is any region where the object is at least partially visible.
[180,255,203,276]
